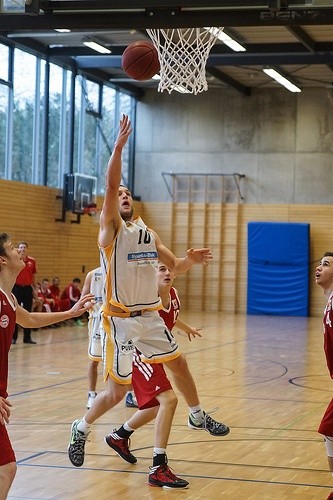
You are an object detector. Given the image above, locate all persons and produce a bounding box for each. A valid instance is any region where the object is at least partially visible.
[68,112,231,467]
[314,251,333,500]
[11,243,204,490]
[0,231,97,500]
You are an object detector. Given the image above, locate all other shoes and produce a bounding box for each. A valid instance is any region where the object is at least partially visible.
[12,338,16,344]
[22,339,37,344]
[326,492,333,500]
[72,319,86,326]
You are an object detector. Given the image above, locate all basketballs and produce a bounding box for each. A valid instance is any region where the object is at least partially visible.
[121,40,161,81]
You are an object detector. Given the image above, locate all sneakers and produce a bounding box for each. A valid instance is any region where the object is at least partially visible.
[187,406,230,436]
[67,418,92,467]
[147,456,190,489]
[125,392,138,408]
[86,392,98,409]
[104,427,138,465]
